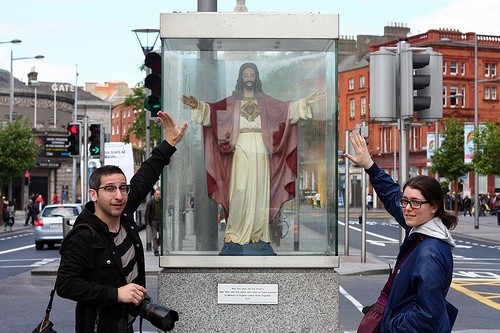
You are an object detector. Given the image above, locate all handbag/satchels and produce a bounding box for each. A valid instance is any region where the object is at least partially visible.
[32,318,58,333]
[357,294,388,333]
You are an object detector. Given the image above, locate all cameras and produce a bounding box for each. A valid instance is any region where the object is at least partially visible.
[129,297,179,331]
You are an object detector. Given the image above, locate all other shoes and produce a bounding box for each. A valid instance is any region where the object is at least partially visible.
[154,252,159,256]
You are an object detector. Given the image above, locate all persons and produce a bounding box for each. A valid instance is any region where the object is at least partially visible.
[55,111,187,333]
[24,194,44,226]
[1,195,16,231]
[53,191,58,203]
[145,190,162,256]
[74,194,80,203]
[445,190,500,225]
[314,192,320,207]
[367,194,372,210]
[182,63,326,246]
[61,191,67,204]
[344,130,456,333]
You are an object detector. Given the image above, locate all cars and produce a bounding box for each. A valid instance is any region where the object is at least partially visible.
[136,186,156,230]
[35,204,84,251]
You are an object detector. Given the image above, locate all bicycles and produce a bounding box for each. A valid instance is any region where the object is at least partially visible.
[282,213,289,238]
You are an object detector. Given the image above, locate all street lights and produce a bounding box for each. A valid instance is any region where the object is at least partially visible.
[131,28,161,249]
[9,49,45,202]
[440,33,479,229]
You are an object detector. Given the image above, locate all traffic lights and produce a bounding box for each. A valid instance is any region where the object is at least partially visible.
[67,122,81,158]
[25,169,29,185]
[89,123,103,158]
[144,52,162,118]
[399,50,431,119]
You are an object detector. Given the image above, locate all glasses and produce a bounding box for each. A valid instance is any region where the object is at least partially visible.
[95,185,130,197]
[398,198,429,210]
